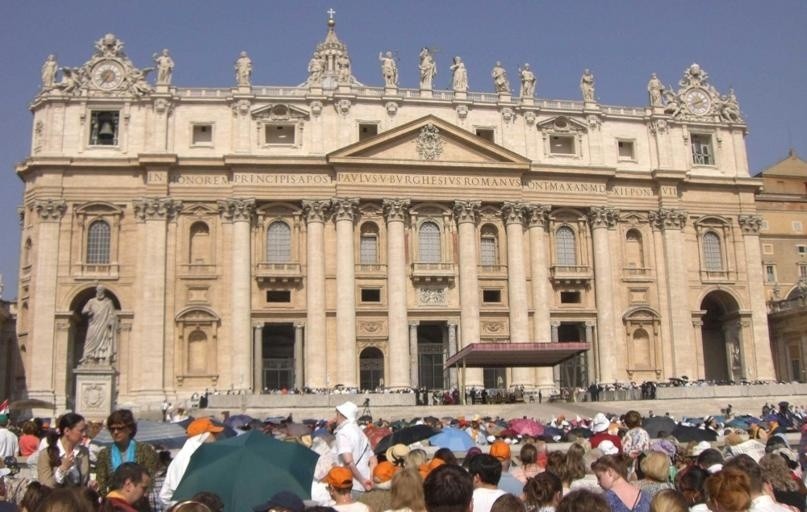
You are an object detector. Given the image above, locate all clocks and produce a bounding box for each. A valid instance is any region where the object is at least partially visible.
[87,56,128,95]
[682,86,712,117]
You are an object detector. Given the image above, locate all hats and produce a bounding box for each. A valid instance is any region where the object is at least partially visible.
[590,413,610,433]
[188,418,224,435]
[322,466,353,489]
[420,459,446,471]
[491,441,512,462]
[374,462,401,484]
[387,444,410,465]
[472,415,481,421]
[0,415,8,425]
[336,401,358,420]
[597,440,619,455]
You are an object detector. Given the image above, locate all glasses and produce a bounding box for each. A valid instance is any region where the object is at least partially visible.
[109,426,127,433]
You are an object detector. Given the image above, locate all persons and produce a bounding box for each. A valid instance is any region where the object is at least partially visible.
[232,51,252,85]
[579,68,594,101]
[417,48,436,85]
[647,73,663,105]
[378,50,396,85]
[516,63,537,96]
[448,56,468,91]
[81,284,115,363]
[152,47,174,84]
[489,60,509,93]
[38,52,58,86]
